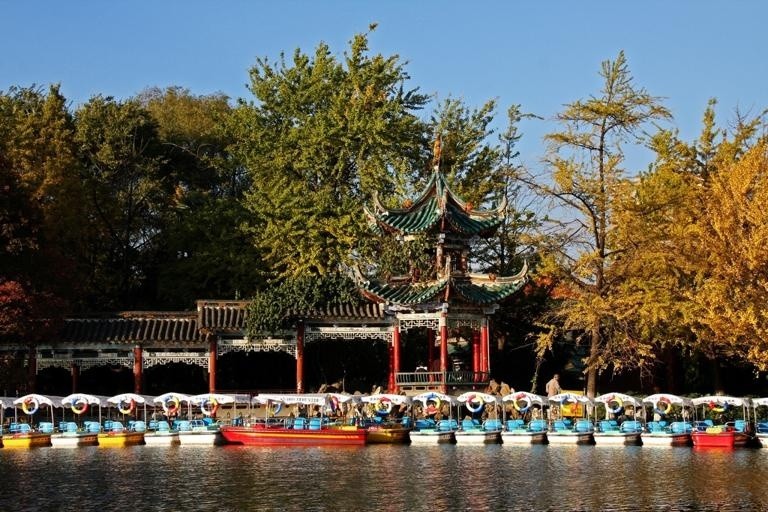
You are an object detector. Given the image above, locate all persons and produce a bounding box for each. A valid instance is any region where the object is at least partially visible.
[451,356,463,371]
[334,377,342,390]
[416,361,428,370]
[547,374,561,420]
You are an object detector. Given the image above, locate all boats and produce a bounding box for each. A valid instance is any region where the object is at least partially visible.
[0,391,768,448]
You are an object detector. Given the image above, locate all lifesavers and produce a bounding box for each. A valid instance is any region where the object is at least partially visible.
[22,397,38,415]
[514,393,532,411]
[709,399,728,412]
[118,395,135,414]
[604,396,623,413]
[423,394,440,411]
[561,394,578,412]
[201,397,218,415]
[265,397,282,414]
[465,394,483,412]
[71,396,88,414]
[375,397,392,414]
[162,395,179,413]
[322,396,338,412]
[653,397,671,414]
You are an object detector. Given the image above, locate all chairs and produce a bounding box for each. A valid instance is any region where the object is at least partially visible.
[574,418,715,435]
[293,417,322,430]
[733,417,766,434]
[401,417,571,434]
[9,418,214,432]
